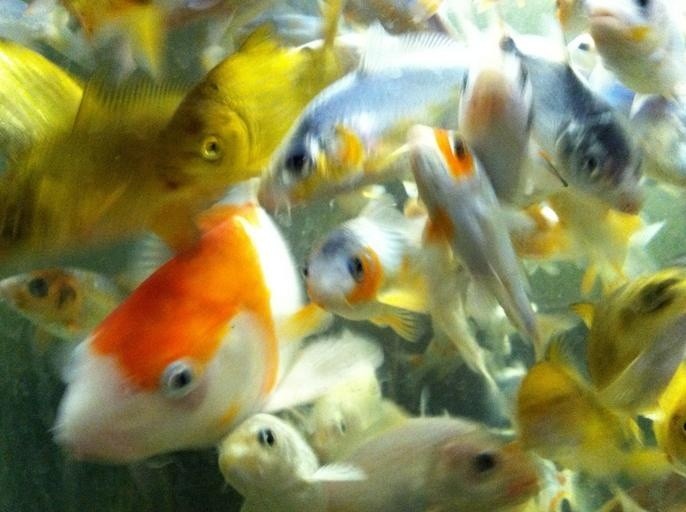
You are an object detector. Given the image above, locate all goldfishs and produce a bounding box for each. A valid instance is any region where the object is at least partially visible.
[0,0,686,512]
